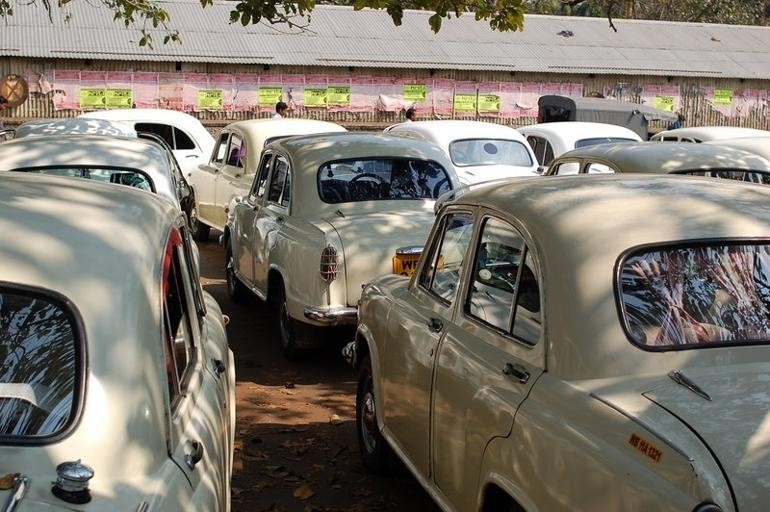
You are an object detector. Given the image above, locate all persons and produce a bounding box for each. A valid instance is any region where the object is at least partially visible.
[272,101,288,118]
[404,107,418,121]
[667,113,687,130]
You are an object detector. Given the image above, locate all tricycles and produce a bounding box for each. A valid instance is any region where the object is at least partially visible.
[537,96,678,139]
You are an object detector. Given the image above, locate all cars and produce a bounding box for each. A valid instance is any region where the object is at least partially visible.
[544,142,770,189]
[384,121,544,188]
[4,132,195,223]
[0,109,770,360]
[342,174,769,508]
[77,110,226,183]
[0,171,235,507]
[189,117,349,250]
[521,123,649,177]
[224,132,468,355]
[655,127,770,161]
